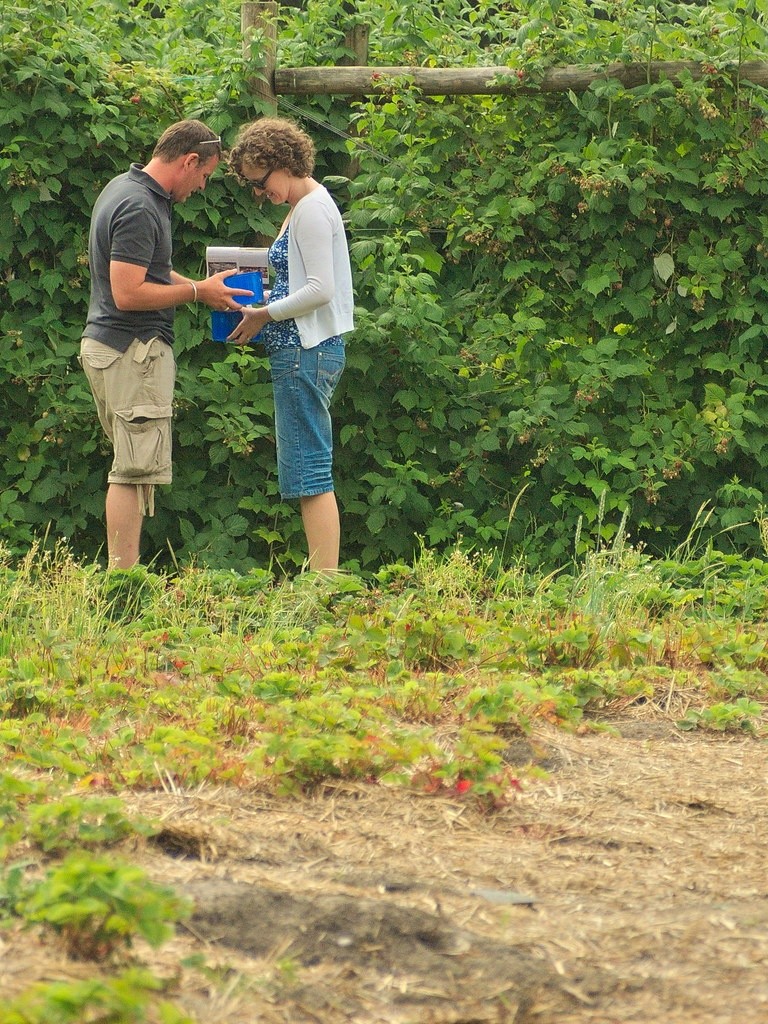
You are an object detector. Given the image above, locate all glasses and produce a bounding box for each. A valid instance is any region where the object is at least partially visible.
[250,167,275,190]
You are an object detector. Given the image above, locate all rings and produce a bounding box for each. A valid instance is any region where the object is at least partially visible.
[224,306,230,312]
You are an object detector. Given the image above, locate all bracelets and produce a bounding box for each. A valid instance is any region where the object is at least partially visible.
[189,282,198,302]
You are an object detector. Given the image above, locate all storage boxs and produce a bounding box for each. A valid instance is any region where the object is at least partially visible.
[222,271,265,308]
[211,310,261,344]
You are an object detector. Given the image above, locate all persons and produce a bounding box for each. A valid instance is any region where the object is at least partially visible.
[77,119,256,568]
[224,117,354,573]
